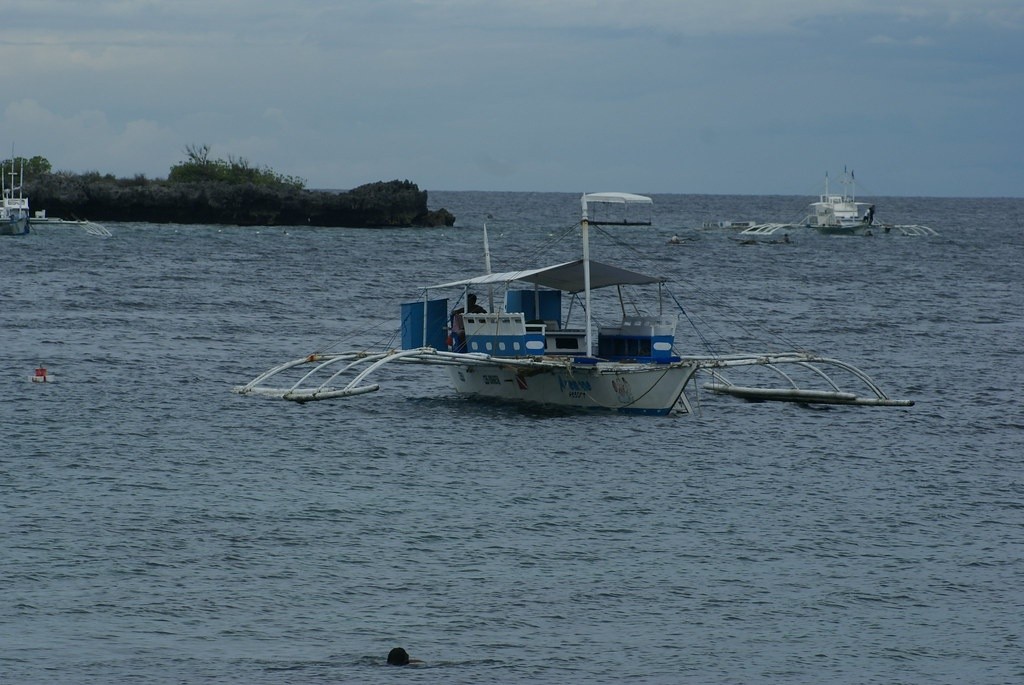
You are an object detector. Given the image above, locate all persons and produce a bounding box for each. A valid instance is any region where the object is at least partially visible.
[863,204,876,225]
[452,293,488,323]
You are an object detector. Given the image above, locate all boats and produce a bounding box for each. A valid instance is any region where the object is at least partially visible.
[0,138,115,239]
[727,162,941,246]
[664,240,689,246]
[231,190,917,417]
